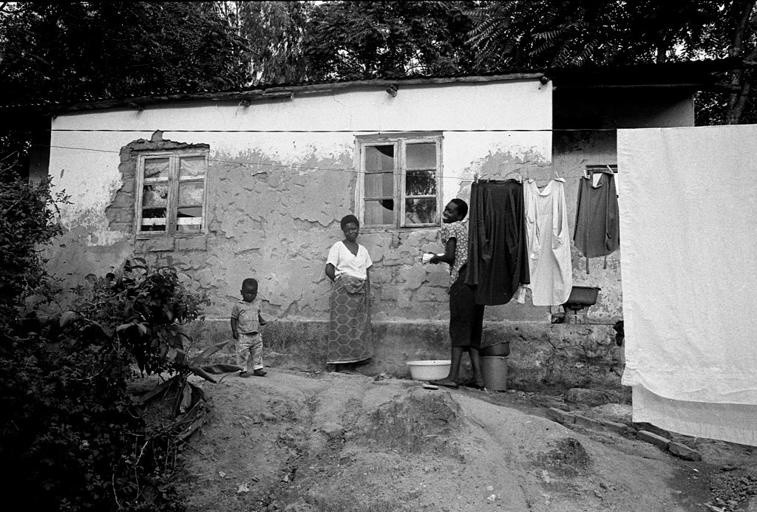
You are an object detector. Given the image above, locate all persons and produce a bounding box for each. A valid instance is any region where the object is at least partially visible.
[325,214,373,373]
[230,278,268,378]
[428,198,486,391]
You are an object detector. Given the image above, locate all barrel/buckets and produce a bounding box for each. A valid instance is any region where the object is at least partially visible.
[479,355,508,392]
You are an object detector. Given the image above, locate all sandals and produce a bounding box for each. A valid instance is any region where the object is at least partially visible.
[429,378,459,388]
[475,381,484,387]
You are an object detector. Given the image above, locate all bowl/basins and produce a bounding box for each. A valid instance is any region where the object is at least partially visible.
[480,341,510,356]
[407,359,452,381]
